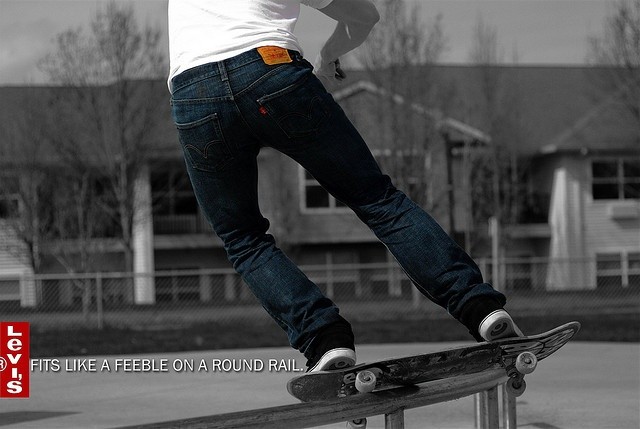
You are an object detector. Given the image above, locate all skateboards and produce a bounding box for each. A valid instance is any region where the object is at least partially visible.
[286,321,580,429]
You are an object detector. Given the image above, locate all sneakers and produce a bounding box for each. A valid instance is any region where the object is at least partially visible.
[462,296,524,342]
[304,322,356,372]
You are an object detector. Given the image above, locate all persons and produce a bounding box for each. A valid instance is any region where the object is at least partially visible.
[167,0,524,374]
